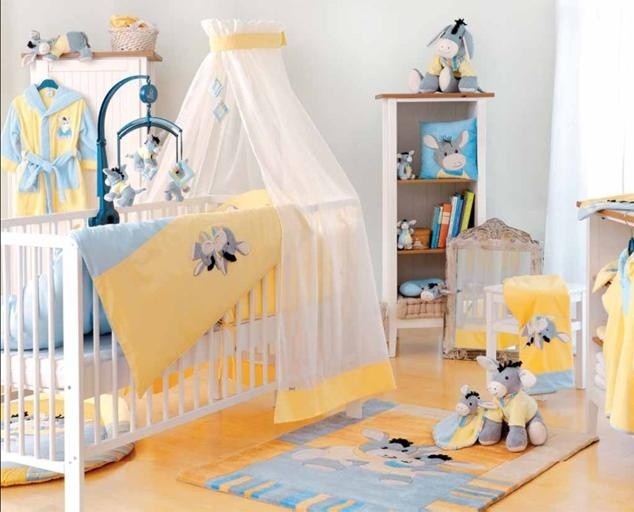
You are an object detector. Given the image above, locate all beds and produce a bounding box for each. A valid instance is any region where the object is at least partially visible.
[1,192,363,511]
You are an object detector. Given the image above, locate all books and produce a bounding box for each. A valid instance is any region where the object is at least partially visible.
[429,189,475,249]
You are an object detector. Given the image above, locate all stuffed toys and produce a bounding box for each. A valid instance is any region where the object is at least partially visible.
[396,218,417,250]
[131,132,161,182]
[417,281,450,302]
[397,149,415,180]
[102,165,146,209]
[163,159,194,202]
[431,383,497,452]
[476,354,548,453]
[407,16,485,95]
[20,30,91,67]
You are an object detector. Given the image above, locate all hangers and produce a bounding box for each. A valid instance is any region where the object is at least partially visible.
[8,62,85,103]
[616,211,634,267]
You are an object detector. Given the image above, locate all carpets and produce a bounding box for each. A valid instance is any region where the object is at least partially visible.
[175,396,601,512]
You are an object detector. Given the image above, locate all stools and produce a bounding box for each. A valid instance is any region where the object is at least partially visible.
[485,284,586,390]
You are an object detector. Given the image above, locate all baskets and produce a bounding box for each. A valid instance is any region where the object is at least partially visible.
[107,20,158,52]
[412,228,432,250]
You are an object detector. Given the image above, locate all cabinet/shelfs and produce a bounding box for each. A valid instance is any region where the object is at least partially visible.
[21,50,164,209]
[373,92,497,358]
[576,193,634,415]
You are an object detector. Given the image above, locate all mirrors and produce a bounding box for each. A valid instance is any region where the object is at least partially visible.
[442,218,544,361]
